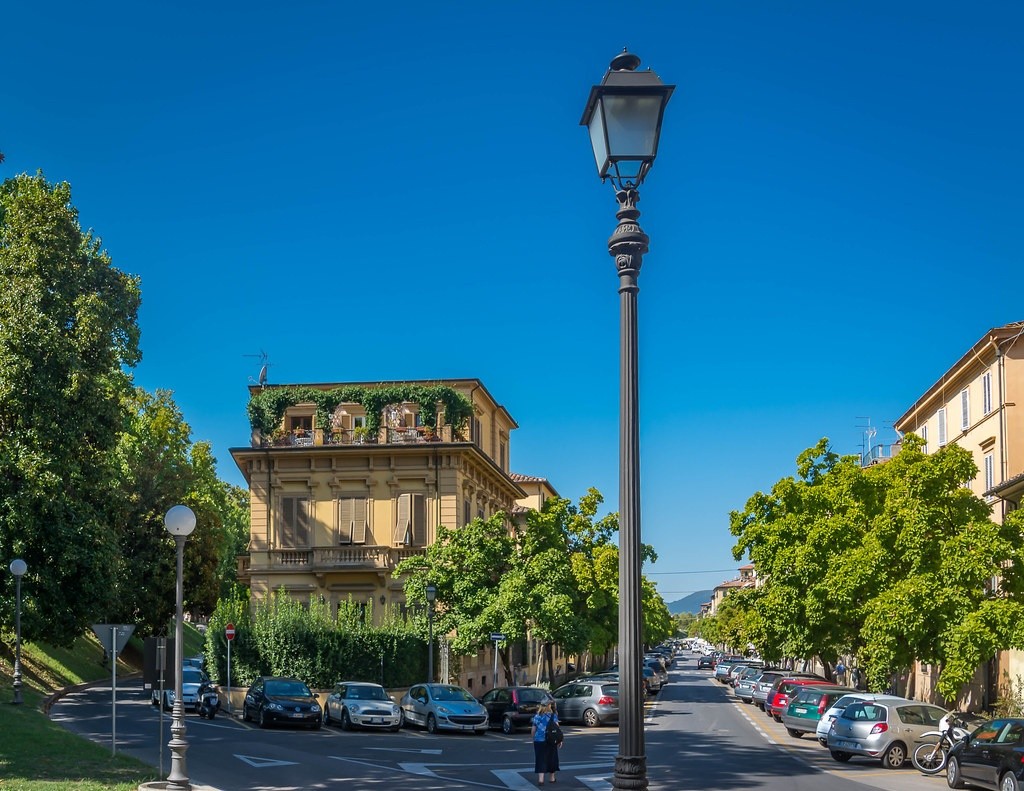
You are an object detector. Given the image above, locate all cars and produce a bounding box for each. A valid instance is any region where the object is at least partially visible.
[243,676,322,731]
[945,717,1024,791]
[151,665,213,713]
[816,693,909,754]
[550,677,620,728]
[324,682,402,733]
[783,691,858,738]
[181,653,205,669]
[400,682,490,736]
[826,699,978,769]
[578,670,647,704]
[592,635,842,724]
[474,686,559,735]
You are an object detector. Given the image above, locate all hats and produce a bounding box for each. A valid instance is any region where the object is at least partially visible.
[541,697,552,707]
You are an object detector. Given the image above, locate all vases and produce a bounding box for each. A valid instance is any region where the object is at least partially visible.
[396,426,407,432]
[416,427,426,431]
[293,428,304,434]
[333,428,344,433]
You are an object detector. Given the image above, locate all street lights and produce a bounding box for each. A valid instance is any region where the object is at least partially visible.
[425,581,440,683]
[163,504,196,791]
[10,559,28,705]
[578,44,679,790]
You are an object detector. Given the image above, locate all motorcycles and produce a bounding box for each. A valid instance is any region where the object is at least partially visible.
[195,689,222,720]
[911,709,996,775]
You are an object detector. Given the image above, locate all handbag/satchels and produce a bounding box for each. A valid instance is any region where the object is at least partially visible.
[832,671,838,677]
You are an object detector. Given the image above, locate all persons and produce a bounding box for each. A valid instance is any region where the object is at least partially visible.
[834,660,846,685]
[532,700,562,785]
[851,665,860,690]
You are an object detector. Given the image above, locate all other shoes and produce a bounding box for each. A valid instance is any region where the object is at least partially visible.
[538,781,546,786]
[549,779,557,784]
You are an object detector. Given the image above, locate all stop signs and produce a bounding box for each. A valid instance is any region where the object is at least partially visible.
[225,623,235,641]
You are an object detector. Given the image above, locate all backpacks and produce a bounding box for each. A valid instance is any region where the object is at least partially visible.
[545,713,564,745]
